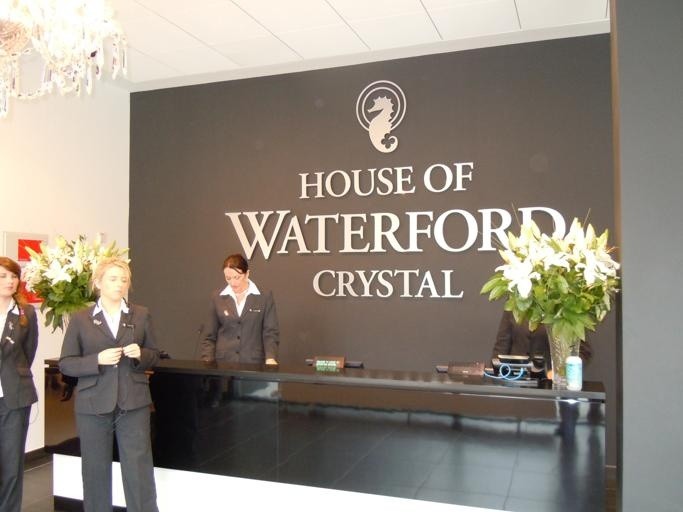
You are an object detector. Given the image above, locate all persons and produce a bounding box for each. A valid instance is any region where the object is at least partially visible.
[0,257,38,512]
[59,257,160,512]
[490,310,593,380]
[197,254,279,364]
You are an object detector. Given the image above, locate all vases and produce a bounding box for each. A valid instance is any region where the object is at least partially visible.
[542,312,583,385]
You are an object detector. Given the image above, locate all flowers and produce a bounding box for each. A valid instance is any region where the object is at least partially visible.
[19,232,135,332]
[479,199,620,377]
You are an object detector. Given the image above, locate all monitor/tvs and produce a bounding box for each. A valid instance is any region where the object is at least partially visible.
[436,365,494,374]
[304,359,364,369]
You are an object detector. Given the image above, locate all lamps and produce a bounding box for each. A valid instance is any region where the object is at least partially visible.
[0,1,127,122]
